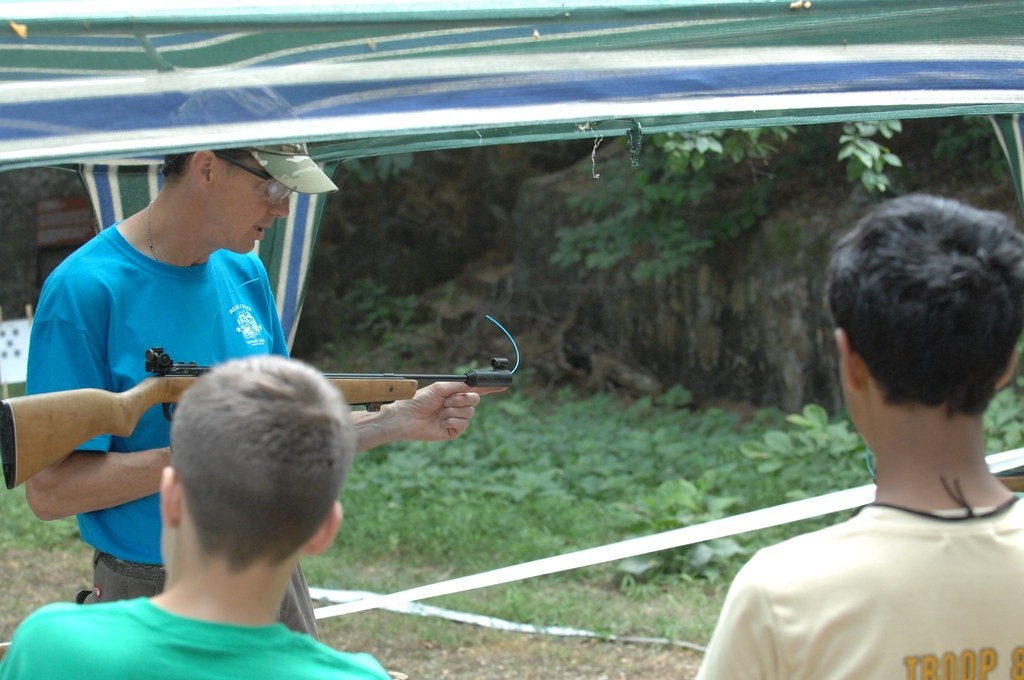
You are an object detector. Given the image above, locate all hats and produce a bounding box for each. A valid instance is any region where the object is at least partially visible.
[172,75,339,194]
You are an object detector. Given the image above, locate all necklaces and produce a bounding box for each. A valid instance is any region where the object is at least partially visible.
[146,201,159,261]
[852,476,1018,523]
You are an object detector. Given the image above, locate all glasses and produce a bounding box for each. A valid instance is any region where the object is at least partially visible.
[215,152,293,202]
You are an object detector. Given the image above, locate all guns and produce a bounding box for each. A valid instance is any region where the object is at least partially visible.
[1,342,515,491]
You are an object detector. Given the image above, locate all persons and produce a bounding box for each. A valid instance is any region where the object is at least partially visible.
[693,193,1024,680]
[25,76,508,637]
[0,355,391,680]
